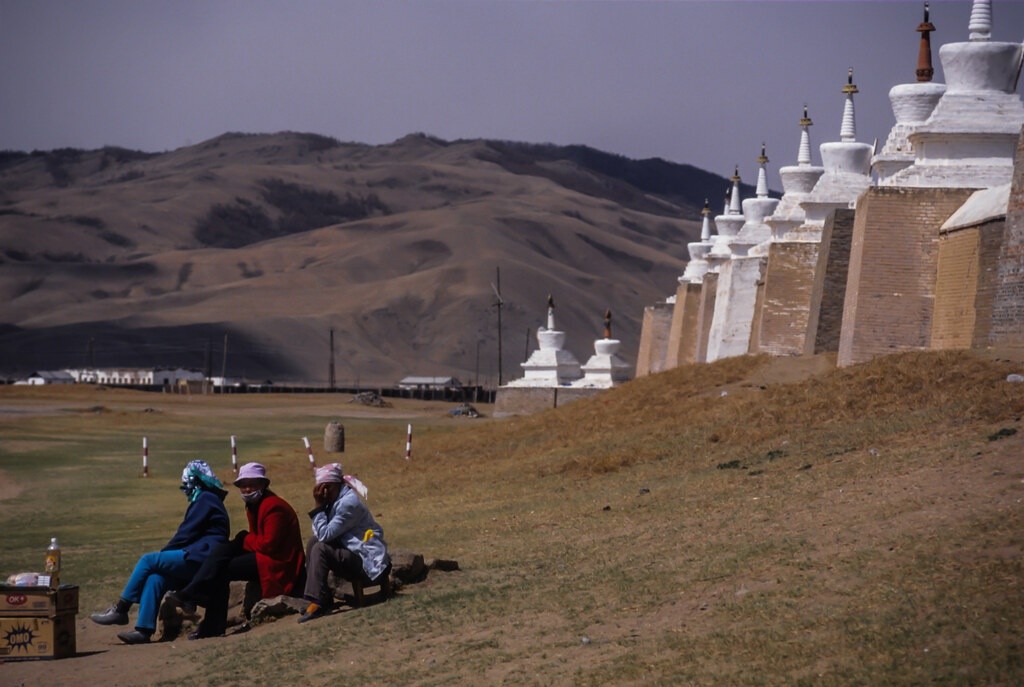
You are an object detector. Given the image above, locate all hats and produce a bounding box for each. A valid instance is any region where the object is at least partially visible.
[233,463,270,489]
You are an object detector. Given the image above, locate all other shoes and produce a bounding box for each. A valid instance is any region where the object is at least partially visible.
[118,632,151,643]
[91,603,128,624]
[166,591,196,615]
[297,597,334,623]
[188,626,225,639]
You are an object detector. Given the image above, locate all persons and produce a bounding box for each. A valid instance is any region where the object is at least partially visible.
[165,462,306,640]
[91,460,230,644]
[298,462,392,622]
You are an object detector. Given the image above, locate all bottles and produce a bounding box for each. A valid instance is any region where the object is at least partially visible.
[45,538,60,587]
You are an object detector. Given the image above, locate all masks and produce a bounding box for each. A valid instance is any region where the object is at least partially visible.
[240,487,263,505]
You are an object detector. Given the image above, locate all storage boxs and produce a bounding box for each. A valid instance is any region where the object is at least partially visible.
[0,582,79,660]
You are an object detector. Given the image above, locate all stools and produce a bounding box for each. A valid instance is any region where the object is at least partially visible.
[346,564,395,601]
